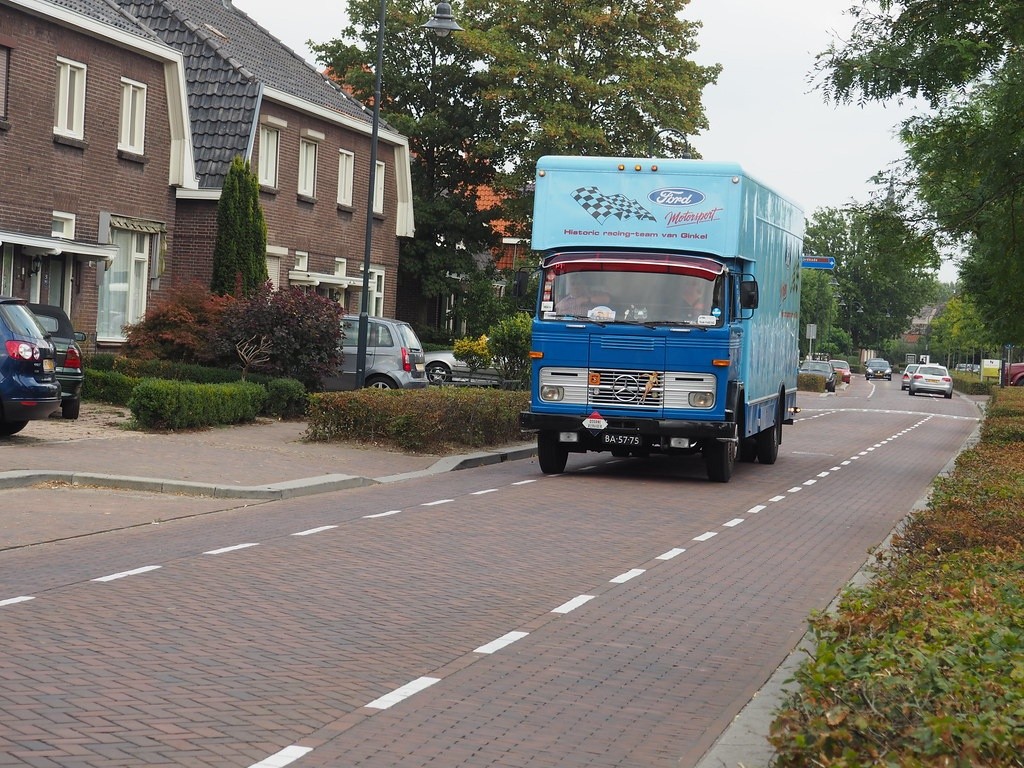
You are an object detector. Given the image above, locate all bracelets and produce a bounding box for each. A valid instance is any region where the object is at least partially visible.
[588,297,591,303]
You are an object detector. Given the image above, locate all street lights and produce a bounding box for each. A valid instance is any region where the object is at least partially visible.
[812,268,846,360]
[355,1,464,391]
[848,302,863,359]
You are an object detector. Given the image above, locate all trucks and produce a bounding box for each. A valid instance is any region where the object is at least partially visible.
[528,159,806,483]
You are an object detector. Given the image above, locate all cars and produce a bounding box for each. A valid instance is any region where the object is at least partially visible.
[828,360,851,384]
[799,360,837,390]
[1004,362,1024,386]
[422,353,511,388]
[0,296,62,436]
[901,364,920,390]
[864,358,892,381]
[313,315,430,392]
[26,303,86,420]
[806,353,831,362]
[909,364,953,399]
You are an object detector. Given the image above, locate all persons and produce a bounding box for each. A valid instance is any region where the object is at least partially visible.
[554,275,613,317]
